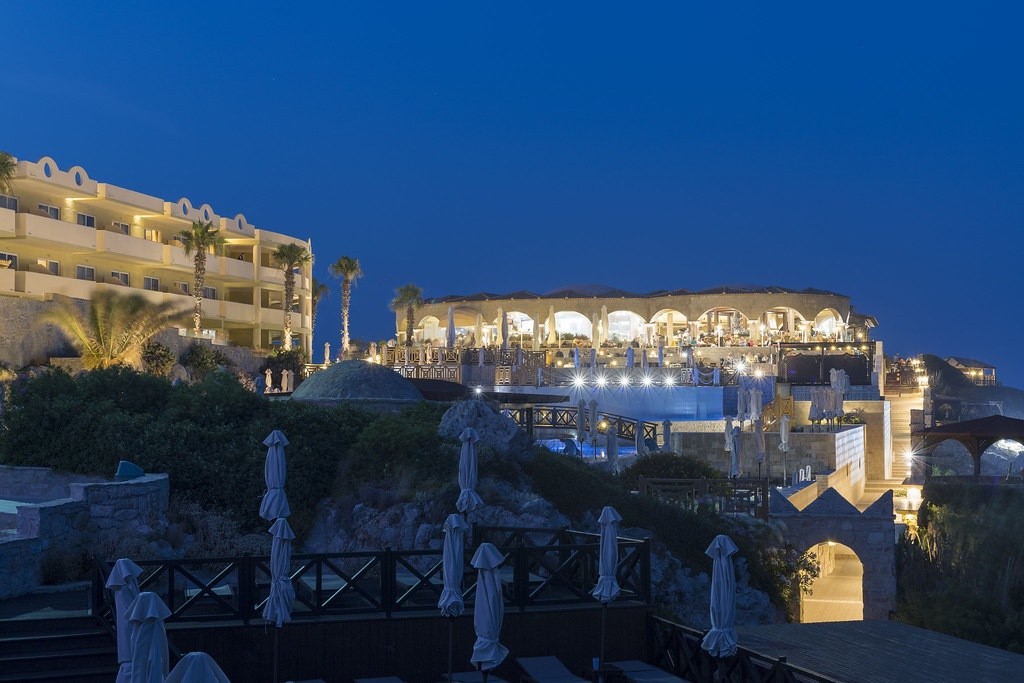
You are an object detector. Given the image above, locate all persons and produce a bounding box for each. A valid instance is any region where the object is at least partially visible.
[686,344,694,366]
[560,439,576,456]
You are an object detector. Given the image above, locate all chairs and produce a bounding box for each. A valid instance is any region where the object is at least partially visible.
[354,676,405,683]
[440,671,509,683]
[396,572,446,606]
[514,655,591,683]
[295,575,362,609]
[494,567,546,599]
[182,584,233,614]
[602,659,688,683]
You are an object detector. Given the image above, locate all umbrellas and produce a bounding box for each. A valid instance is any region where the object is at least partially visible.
[163,651,230,683]
[755,428,764,484]
[123,591,172,683]
[699,534,741,683]
[735,386,763,431]
[607,422,620,477]
[260,301,665,394]
[264,517,294,630]
[578,399,600,464]
[455,427,485,513]
[779,413,791,487]
[636,418,646,493]
[723,413,736,469]
[468,543,511,683]
[587,504,625,683]
[808,369,848,431]
[104,556,144,683]
[437,513,467,682]
[260,429,296,521]
[730,426,743,507]
[661,419,672,453]
